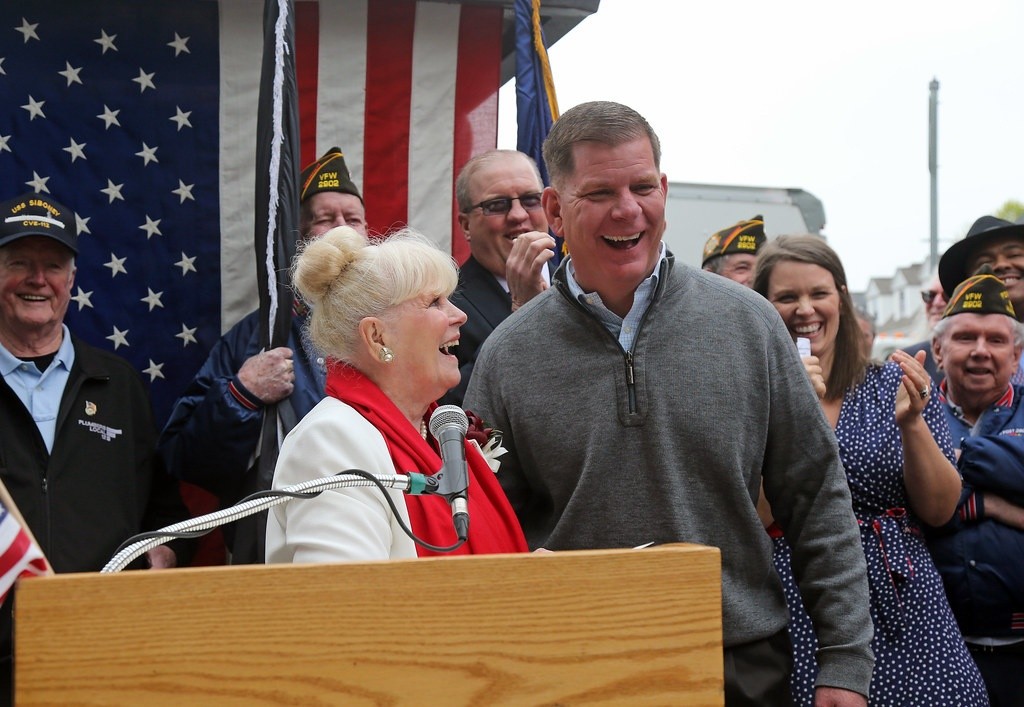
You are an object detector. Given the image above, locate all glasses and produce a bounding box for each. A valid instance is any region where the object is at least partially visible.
[462,192,542,217]
[921,290,949,304]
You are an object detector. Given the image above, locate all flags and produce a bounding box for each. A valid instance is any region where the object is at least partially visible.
[232,0,301,564]
[516,0,569,284]
[0,505,54,607]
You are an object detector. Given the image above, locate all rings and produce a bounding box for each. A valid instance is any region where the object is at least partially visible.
[920,385,928,398]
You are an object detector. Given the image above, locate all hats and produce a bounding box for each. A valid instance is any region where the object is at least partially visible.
[300,147,364,206]
[938,216,1024,297]
[0,193,79,253]
[941,275,1018,320]
[701,214,767,269]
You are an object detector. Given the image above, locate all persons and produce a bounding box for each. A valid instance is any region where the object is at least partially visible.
[0,194,178,571]
[438,150,559,406]
[152,143,367,566]
[264,221,553,565]
[699,215,1024,707]
[463,102,875,707]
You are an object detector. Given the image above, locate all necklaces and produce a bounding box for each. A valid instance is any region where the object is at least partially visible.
[420,420,427,440]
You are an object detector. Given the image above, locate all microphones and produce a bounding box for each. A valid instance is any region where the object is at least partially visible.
[428,404,470,541]
